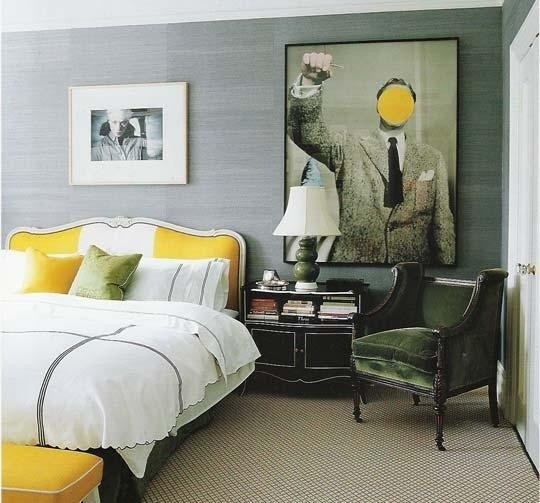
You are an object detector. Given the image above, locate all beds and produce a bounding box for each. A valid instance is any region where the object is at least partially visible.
[1,215,265,502]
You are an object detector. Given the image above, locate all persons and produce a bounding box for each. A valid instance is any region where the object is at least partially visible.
[92,109,150,161]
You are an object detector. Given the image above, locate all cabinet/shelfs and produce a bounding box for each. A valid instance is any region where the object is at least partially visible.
[239,278,371,405]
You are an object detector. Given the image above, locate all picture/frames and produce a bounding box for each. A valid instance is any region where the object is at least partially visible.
[68,80,188,185]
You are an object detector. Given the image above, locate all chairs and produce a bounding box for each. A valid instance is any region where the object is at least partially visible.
[345,259,509,451]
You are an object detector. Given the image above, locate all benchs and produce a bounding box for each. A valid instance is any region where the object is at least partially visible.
[1,440,105,502]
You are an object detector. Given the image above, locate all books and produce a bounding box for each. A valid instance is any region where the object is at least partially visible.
[247,296,358,321]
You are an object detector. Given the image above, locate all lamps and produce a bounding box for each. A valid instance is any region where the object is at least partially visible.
[272,185,344,290]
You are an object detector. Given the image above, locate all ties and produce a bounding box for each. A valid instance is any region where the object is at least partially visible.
[388,136,404,203]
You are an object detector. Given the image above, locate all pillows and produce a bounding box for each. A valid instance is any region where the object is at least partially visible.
[66,243,142,300]
[207,257,231,308]
[14,245,86,293]
[122,259,226,313]
